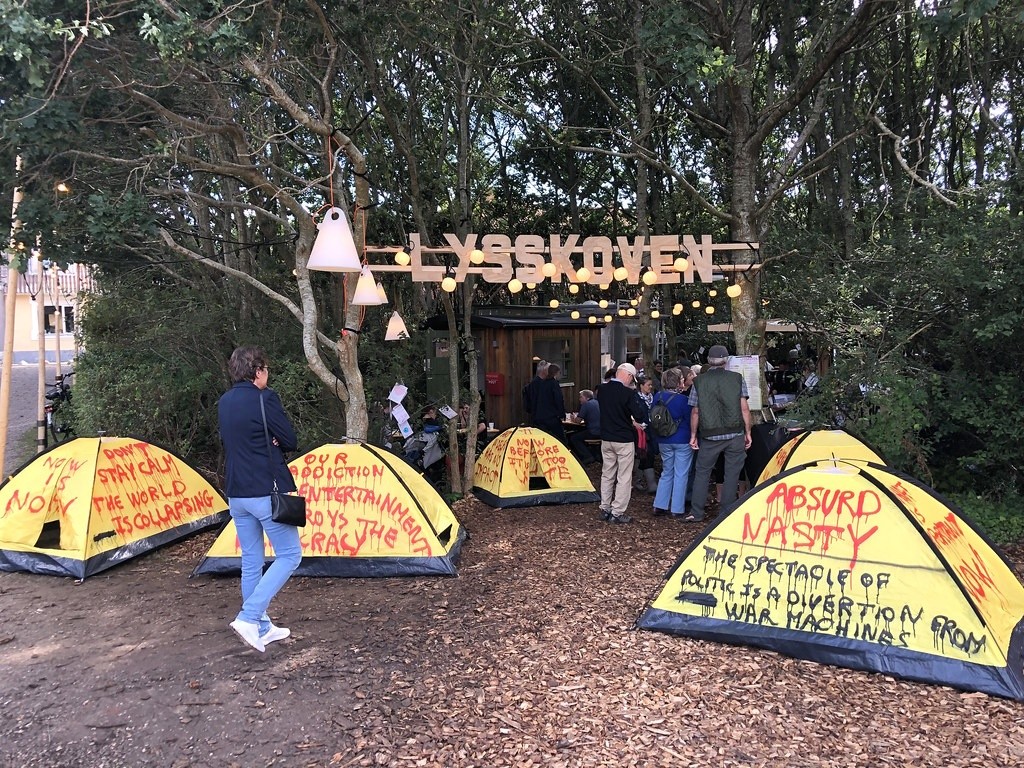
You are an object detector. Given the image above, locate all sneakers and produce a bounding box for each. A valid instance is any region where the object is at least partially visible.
[229,617,266,653]
[599,510,633,523]
[260,622,291,646]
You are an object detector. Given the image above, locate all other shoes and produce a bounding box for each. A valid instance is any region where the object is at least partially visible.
[669,512,688,517]
[683,512,702,522]
[652,507,667,515]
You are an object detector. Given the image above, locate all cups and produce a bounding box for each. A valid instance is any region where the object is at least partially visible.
[489,423,495,430]
[566,414,572,421]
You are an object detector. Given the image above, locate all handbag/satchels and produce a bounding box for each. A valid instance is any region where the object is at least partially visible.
[270,491,306,527]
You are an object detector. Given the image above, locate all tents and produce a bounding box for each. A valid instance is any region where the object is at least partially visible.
[470,423,603,510]
[189,437,471,577]
[753,423,893,490]
[2,430,232,583]
[632,458,1024,703]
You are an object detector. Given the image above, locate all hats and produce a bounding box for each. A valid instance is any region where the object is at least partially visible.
[708,345,729,358]
[618,362,638,383]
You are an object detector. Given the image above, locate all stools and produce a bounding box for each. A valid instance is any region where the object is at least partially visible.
[584,439,603,464]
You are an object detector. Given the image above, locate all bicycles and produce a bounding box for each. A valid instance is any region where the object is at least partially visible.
[42,371,77,444]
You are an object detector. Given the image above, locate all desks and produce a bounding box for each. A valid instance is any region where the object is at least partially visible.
[562,419,585,444]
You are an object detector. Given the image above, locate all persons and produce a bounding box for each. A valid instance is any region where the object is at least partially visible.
[677,346,753,523]
[523,361,551,426]
[624,356,712,406]
[568,389,602,465]
[218,345,303,653]
[632,376,658,495]
[650,368,693,520]
[535,364,567,444]
[597,362,648,524]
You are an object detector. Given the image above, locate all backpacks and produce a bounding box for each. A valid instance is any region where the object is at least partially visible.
[650,391,683,439]
